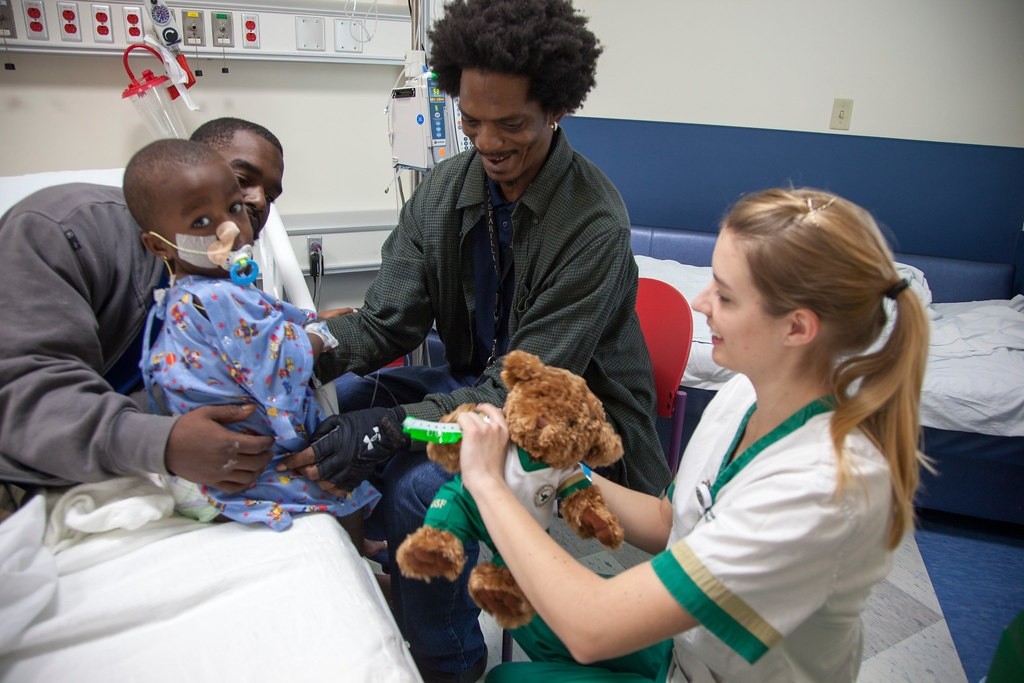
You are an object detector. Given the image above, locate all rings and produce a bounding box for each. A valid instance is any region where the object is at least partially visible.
[483,416,491,423]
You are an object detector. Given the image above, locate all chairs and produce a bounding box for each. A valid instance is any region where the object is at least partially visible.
[501,278,693,662]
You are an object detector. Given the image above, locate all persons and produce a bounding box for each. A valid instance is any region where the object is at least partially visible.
[277,0,669,683]
[124,139,403,601]
[0,118,283,492]
[457,188,927,683]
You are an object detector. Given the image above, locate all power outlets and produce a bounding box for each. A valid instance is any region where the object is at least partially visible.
[56,2,82,42]
[122,7,145,44]
[241,13,260,48]
[21,0,49,40]
[91,4,114,44]
[307,236,322,266]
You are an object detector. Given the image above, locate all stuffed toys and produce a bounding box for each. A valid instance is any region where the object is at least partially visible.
[395,350,624,630]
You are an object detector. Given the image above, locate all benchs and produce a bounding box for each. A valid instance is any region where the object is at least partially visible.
[421,223,1024,526]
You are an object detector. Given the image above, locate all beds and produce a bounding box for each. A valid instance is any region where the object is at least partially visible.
[1,168,430,683]
